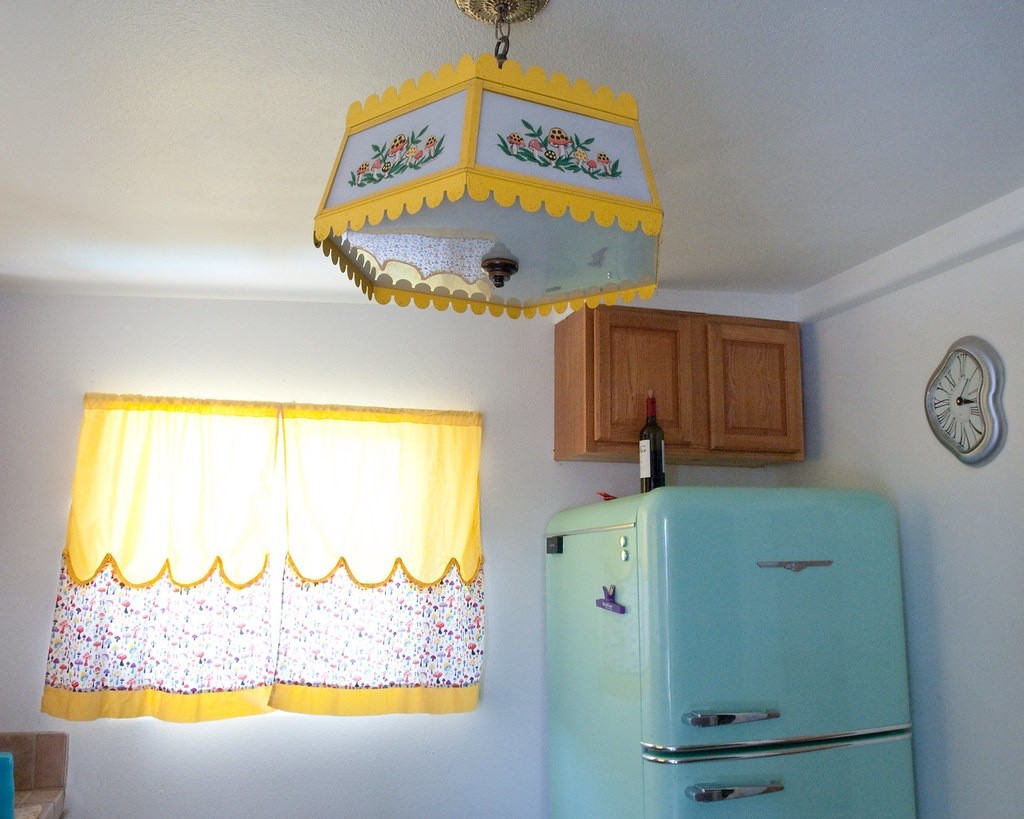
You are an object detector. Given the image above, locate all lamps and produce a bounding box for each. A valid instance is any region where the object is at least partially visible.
[309,0,665,321]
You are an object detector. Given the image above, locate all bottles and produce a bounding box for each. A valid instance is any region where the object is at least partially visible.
[638,389,669,496]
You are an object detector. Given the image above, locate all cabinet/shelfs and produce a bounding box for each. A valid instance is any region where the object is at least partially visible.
[553,299,807,468]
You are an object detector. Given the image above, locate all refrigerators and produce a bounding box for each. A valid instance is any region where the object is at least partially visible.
[540,478,918,819]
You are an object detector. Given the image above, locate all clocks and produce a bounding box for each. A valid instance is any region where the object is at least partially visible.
[924,336,1005,466]
